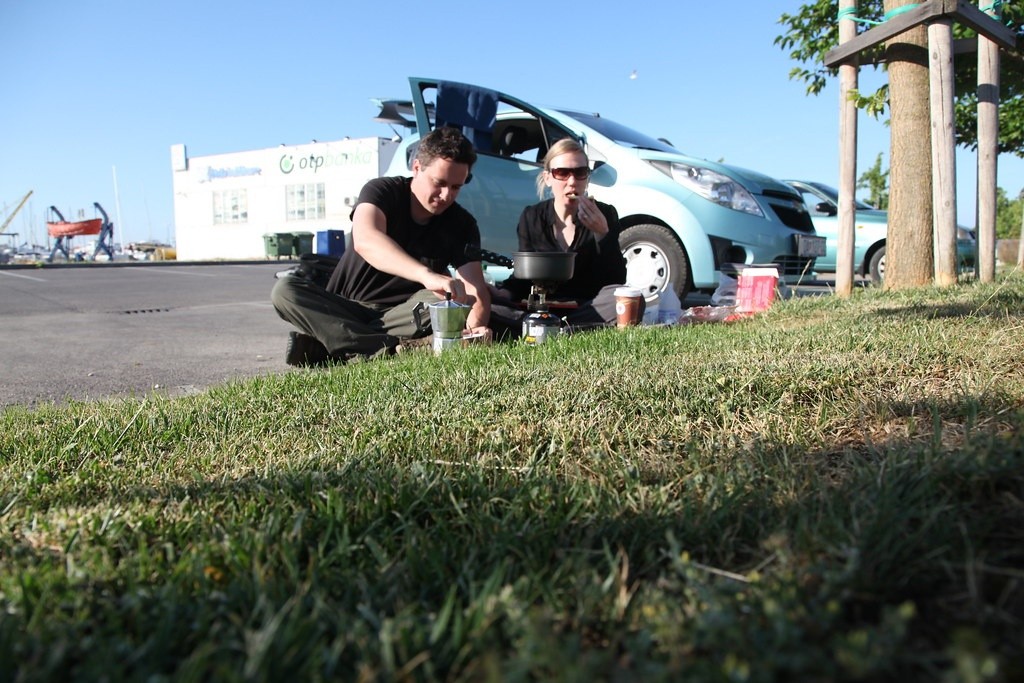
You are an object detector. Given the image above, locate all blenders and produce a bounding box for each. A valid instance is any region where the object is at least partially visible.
[413,292,472,360]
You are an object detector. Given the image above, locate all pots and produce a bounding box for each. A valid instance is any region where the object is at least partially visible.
[511,250,577,281]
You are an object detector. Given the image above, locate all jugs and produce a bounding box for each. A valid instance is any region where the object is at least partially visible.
[657,283,681,324]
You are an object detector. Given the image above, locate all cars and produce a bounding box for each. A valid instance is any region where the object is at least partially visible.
[786,174,978,287]
[369,75,831,308]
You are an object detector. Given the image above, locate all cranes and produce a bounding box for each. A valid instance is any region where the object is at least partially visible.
[0,190,35,233]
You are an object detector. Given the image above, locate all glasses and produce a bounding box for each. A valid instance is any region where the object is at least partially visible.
[547,165,594,179]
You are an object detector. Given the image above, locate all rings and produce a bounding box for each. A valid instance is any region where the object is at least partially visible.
[584,214,588,219]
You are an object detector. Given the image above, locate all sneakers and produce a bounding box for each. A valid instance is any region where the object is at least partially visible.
[284,330,328,367]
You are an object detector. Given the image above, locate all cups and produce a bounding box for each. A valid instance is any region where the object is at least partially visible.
[462,332,485,352]
[614,286,642,330]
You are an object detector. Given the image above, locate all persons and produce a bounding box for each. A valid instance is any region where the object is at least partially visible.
[271,126,492,368]
[486,138,645,341]
